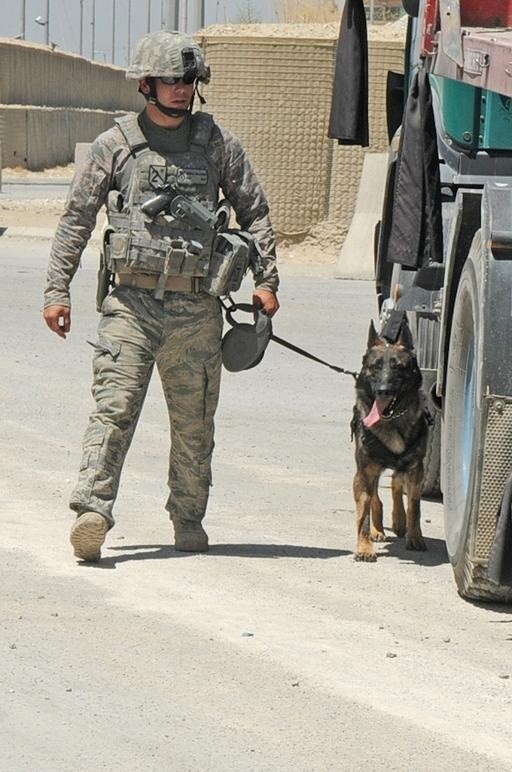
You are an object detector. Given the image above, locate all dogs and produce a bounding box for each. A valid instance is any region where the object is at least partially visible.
[346,310,432,563]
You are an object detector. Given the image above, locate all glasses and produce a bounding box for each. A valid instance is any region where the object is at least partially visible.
[160,78,195,84]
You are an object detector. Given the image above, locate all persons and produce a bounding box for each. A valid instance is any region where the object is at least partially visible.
[43,26,282,565]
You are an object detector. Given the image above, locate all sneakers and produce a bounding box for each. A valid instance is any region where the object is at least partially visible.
[172,516,208,552]
[70,509,107,564]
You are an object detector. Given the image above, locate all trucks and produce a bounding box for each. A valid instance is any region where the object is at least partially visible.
[374,1,512,608]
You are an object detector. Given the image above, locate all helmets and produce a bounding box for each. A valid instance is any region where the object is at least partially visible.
[126,32,210,80]
